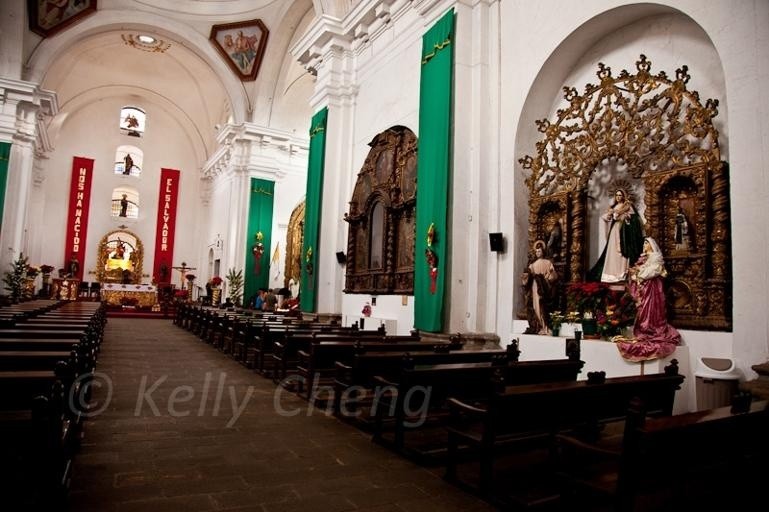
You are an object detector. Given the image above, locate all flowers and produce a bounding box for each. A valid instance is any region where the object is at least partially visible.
[24,265,42,277]
[185,273,197,282]
[548,277,630,340]
[39,263,56,275]
[209,276,223,286]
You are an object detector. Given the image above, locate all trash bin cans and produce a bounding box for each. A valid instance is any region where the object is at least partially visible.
[693,356,741,413]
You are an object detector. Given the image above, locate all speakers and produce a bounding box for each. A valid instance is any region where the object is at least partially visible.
[489,232,503,251]
[336,251,345,263]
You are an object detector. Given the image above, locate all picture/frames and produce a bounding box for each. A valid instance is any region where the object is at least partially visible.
[24,1,99,40]
[208,19,270,83]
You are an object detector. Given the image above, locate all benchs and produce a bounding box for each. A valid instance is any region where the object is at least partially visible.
[171,299,769,512]
[1,297,108,511]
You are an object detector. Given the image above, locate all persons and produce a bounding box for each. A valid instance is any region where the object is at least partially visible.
[588,189,641,283]
[521,240,558,335]
[159,259,168,282]
[123,154,133,172]
[176,268,193,290]
[119,194,128,215]
[234,30,251,73]
[612,237,680,363]
[220,298,234,309]
[674,205,693,250]
[50,280,78,301]
[543,216,562,262]
[250,288,277,312]
[67,255,79,278]
